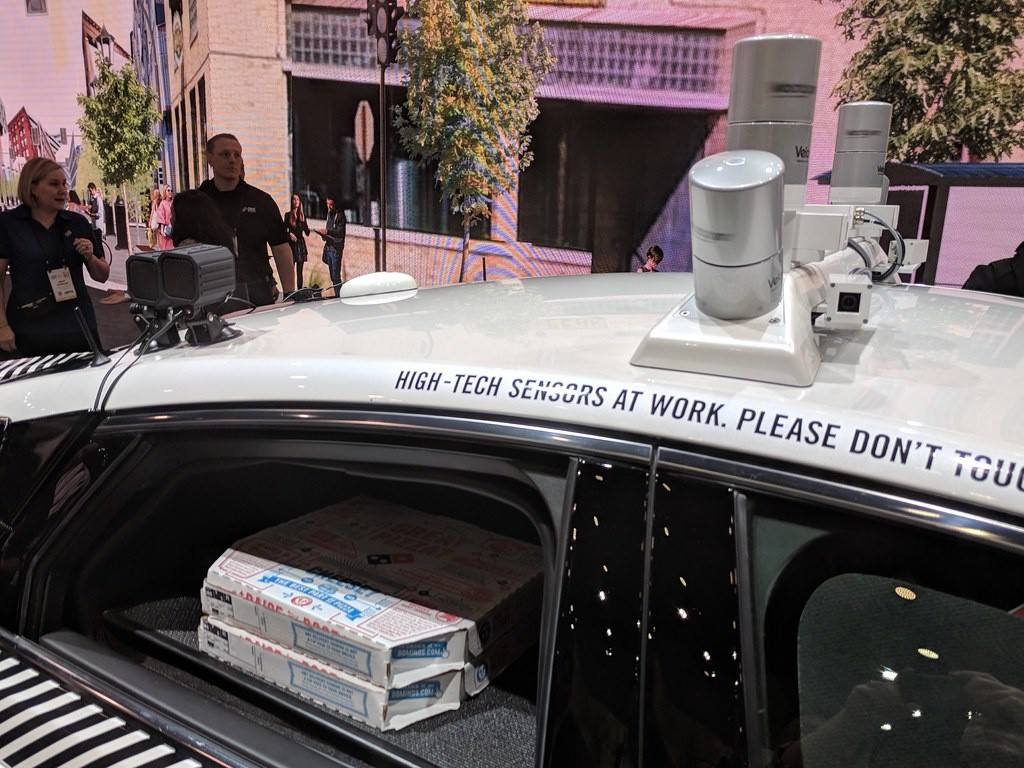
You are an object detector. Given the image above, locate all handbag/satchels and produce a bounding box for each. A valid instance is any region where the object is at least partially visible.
[164,226,173,237]
[90,196,98,213]
[151,210,159,230]
[145,227,156,248]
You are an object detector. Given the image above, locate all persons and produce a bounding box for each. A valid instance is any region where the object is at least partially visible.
[636,246,662,272]
[0,157,111,353]
[962,237,1024,299]
[169,189,235,253]
[68,179,174,253]
[314,194,347,296]
[172,133,294,306]
[285,193,310,291]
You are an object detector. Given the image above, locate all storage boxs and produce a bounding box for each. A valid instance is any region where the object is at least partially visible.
[197,608,542,734]
[199,499,547,691]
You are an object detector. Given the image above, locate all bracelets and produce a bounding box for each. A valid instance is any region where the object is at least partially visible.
[1,323,9,329]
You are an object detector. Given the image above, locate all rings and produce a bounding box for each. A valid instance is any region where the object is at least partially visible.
[85,246,88,249]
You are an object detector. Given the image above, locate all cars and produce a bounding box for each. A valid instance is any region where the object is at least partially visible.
[0,36,1024,767]
